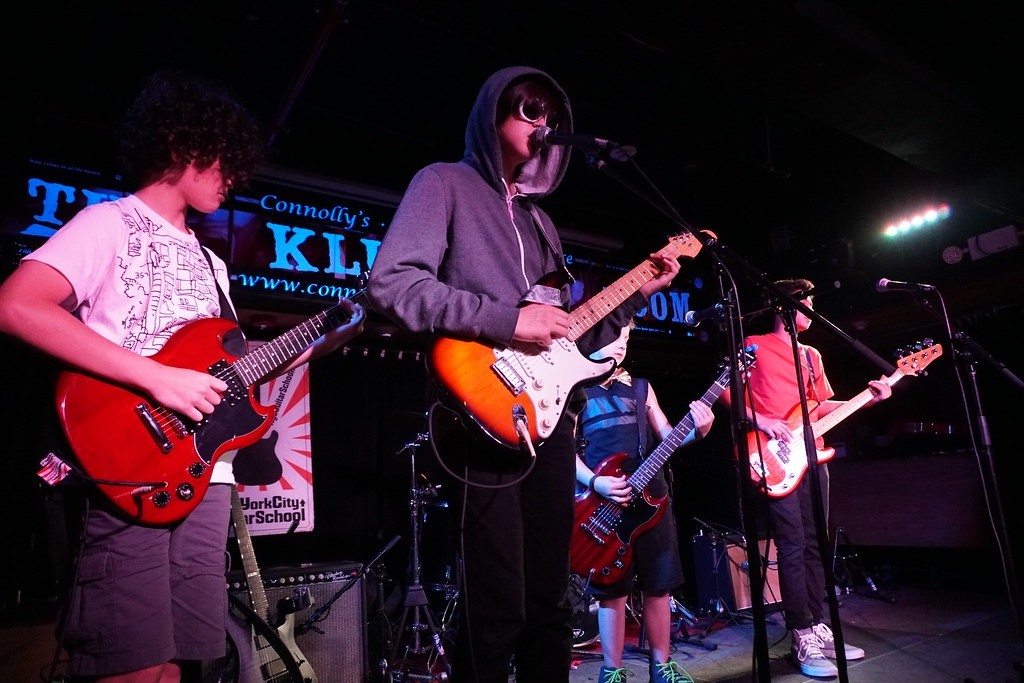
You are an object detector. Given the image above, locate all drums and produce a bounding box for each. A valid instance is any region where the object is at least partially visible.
[567,572,603,648]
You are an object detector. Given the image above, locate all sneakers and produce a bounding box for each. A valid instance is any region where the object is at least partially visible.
[572,610,600,649]
[791,628,838,677]
[648,657,694,683]
[598,666,634,683]
[812,621,865,660]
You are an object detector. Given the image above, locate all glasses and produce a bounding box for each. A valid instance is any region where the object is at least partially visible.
[518,98,560,131]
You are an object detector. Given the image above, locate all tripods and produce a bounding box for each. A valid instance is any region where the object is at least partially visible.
[383,420,453,681]
[692,517,778,639]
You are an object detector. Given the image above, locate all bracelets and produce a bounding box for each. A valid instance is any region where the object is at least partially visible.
[589,475,599,492]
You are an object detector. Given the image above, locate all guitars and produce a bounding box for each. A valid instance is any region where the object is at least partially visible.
[427,230,720,453]
[733,338,944,498]
[569,341,759,588]
[53,282,373,530]
[231,490,319,683]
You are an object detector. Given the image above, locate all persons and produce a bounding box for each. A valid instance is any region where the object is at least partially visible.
[713,279,893,677]
[0,93,347,683]
[363,65,681,683]
[574,315,715,683]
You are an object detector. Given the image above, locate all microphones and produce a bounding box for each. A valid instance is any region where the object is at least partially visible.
[740,561,778,570]
[876,278,936,292]
[531,126,619,151]
[685,303,723,328]
[589,604,598,614]
[757,481,773,490]
[295,624,325,635]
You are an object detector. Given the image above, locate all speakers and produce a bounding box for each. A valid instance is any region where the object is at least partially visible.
[694,531,784,617]
[223,559,369,683]
